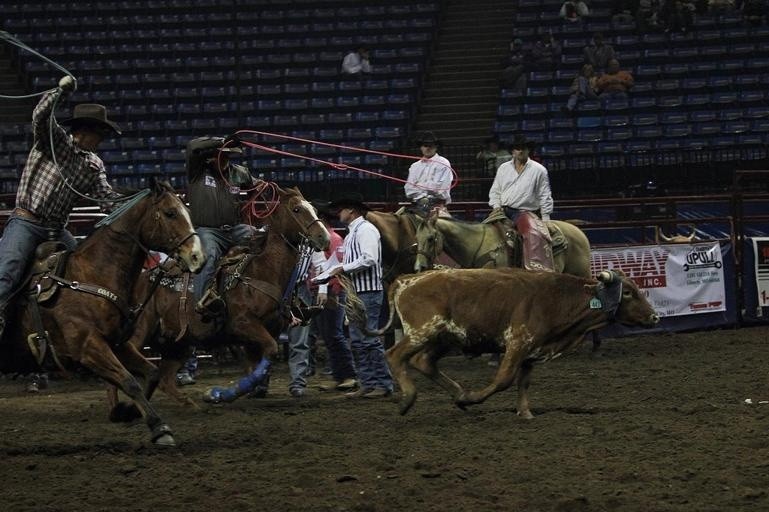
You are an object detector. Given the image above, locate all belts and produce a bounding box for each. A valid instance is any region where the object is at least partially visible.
[14,208,40,221]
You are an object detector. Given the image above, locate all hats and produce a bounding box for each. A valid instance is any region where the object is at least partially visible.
[328,191,371,212]
[508,135,535,155]
[484,135,505,149]
[411,129,439,145]
[60,103,122,137]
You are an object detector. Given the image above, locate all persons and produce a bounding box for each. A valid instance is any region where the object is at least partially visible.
[488,132,557,272]
[250,244,328,398]
[475,134,502,176]
[402,129,455,217]
[2,73,124,340]
[184,134,262,314]
[175,351,200,386]
[340,42,371,73]
[328,191,395,399]
[528,141,547,166]
[304,198,358,391]
[499,0,768,116]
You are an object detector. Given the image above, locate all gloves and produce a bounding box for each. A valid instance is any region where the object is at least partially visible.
[59,74,75,92]
[225,134,240,147]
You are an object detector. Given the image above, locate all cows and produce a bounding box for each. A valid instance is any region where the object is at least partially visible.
[336,262,660,420]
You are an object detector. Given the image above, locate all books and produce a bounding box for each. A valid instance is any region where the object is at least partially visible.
[309,262,344,284]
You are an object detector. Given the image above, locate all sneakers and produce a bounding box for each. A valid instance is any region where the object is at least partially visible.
[291,390,304,397]
[176,372,195,385]
[336,378,389,399]
[247,390,266,399]
[304,367,333,376]
[195,297,226,312]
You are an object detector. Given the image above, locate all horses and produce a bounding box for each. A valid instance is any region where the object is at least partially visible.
[414,208,602,367]
[331,203,452,350]
[0,173,207,447]
[113,186,331,410]
